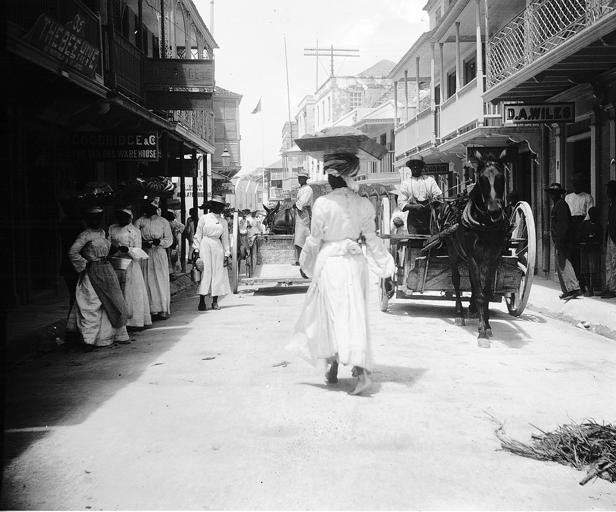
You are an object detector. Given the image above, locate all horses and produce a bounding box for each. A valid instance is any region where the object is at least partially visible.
[438,144,513,349]
[262,200,296,234]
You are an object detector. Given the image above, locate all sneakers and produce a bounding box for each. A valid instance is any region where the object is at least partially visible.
[559,290,581,300]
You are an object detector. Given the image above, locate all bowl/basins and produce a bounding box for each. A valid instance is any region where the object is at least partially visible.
[110,255,132,270]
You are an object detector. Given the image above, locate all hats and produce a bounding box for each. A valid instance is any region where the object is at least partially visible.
[544,183,567,194]
[210,195,230,207]
[406,155,424,167]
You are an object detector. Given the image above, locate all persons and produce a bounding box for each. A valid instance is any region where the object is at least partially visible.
[293,172,315,266]
[165,207,270,281]
[391,154,444,248]
[567,179,594,287]
[585,206,604,295]
[109,205,153,328]
[190,194,233,312]
[66,203,131,348]
[293,154,400,396]
[543,180,584,300]
[601,181,616,298]
[134,196,174,320]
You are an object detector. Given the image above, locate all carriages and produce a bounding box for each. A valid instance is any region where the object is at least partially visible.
[383,151,540,348]
[231,200,317,294]
